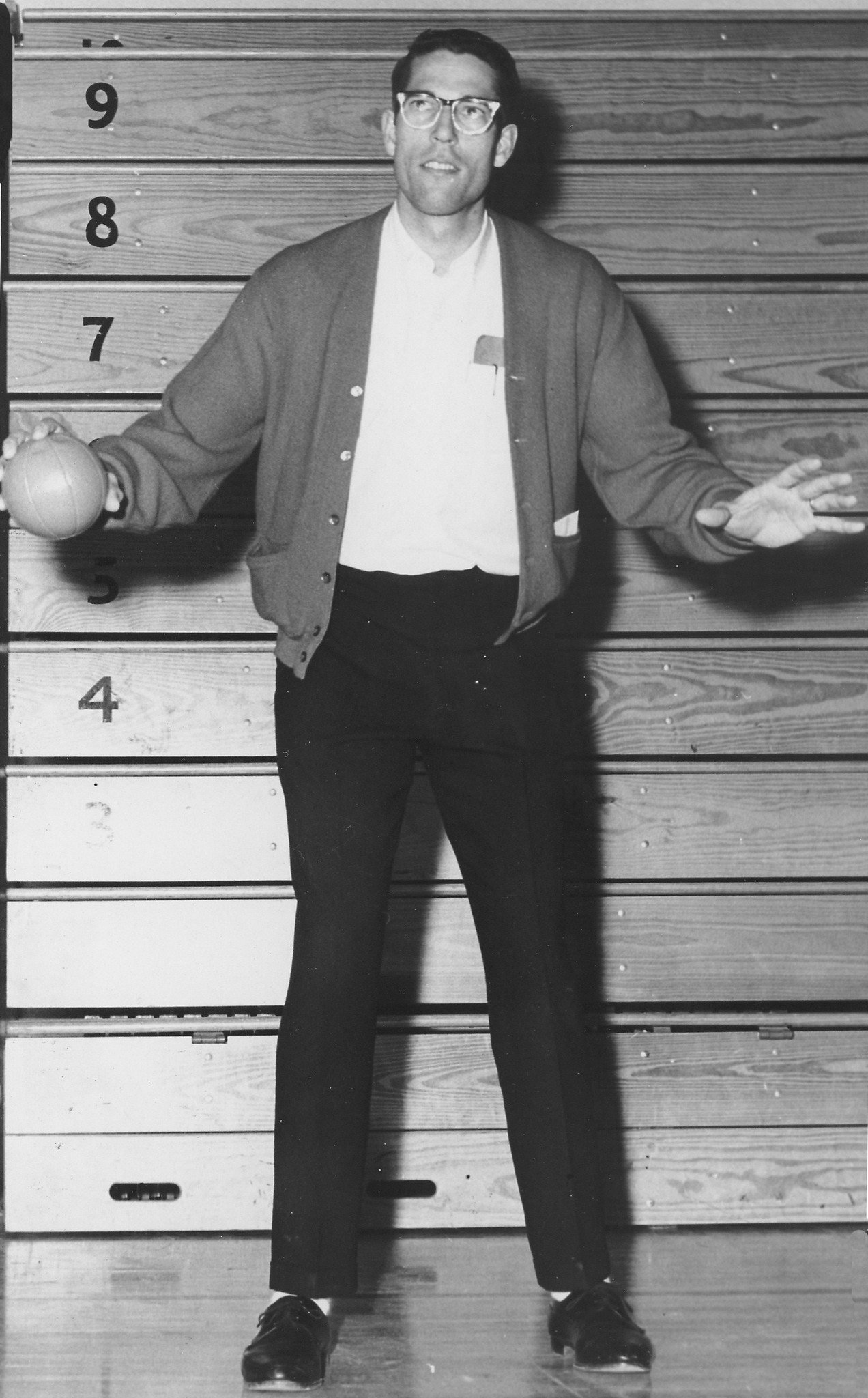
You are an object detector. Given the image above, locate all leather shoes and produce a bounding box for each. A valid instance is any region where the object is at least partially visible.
[241,1296,329,1392]
[546,1279,654,1375]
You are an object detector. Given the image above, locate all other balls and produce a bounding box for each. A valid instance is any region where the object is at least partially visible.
[4,435,109,540]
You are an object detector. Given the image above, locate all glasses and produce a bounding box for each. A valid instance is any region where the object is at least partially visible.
[394,90,506,136]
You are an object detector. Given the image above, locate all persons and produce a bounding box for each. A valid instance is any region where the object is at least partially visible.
[0,28,863,1395]
